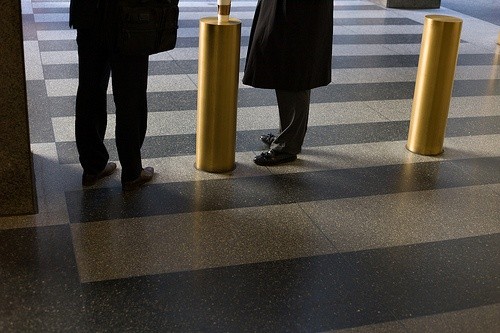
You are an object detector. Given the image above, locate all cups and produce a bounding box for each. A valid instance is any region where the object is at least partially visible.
[217,0,232,22]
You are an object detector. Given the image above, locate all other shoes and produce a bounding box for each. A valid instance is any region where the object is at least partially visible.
[253,151,297,166]
[259,133,276,146]
[121,167,154,191]
[81,162,116,187]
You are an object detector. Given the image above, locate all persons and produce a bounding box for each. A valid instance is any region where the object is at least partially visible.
[66,0,178,187]
[243,1,334,167]
